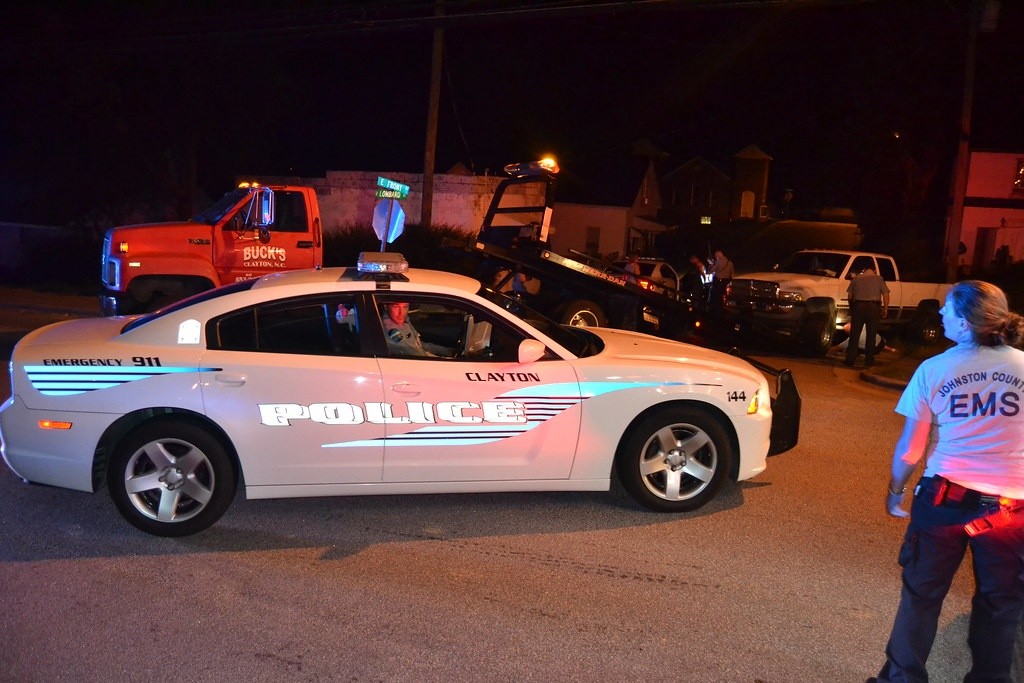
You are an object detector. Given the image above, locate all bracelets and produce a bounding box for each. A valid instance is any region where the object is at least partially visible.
[889,481,907,494]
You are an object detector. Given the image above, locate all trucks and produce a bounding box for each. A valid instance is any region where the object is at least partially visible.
[103,176,634,320]
[723,247,967,358]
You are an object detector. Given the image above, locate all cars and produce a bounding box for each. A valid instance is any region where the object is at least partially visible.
[0,253,777,538]
[602,259,691,288]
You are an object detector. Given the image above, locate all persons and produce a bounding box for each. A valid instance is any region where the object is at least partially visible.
[688,251,733,304]
[625,253,640,275]
[865,280,1024,683]
[843,260,890,365]
[381,302,449,359]
